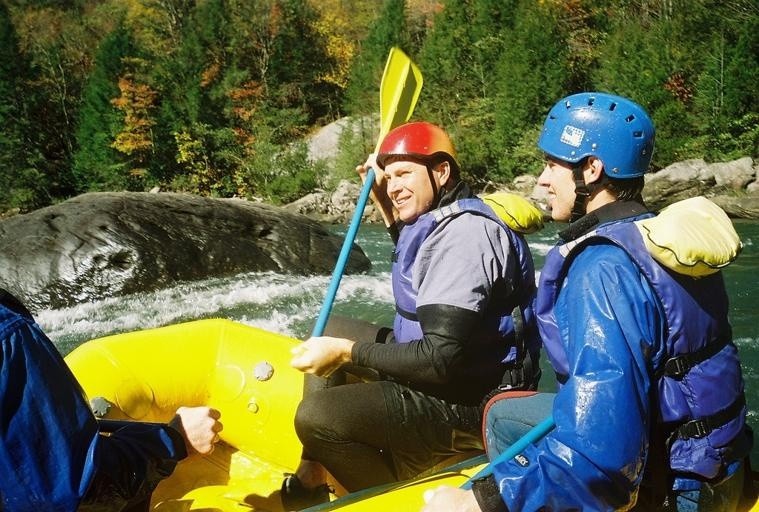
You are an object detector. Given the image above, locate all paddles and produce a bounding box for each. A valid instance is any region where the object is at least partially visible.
[312,46,424,336]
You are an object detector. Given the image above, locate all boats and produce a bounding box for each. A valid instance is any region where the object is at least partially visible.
[62,314,759,511]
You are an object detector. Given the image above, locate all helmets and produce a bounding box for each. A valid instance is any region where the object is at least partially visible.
[538,92,655,178]
[374,121,461,171]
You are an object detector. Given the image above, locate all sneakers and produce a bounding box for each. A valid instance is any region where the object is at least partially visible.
[241,473,330,512]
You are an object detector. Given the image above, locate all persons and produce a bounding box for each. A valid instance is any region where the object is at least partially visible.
[0,285,225,512]
[420,91,750,511]
[282,121,541,511]
